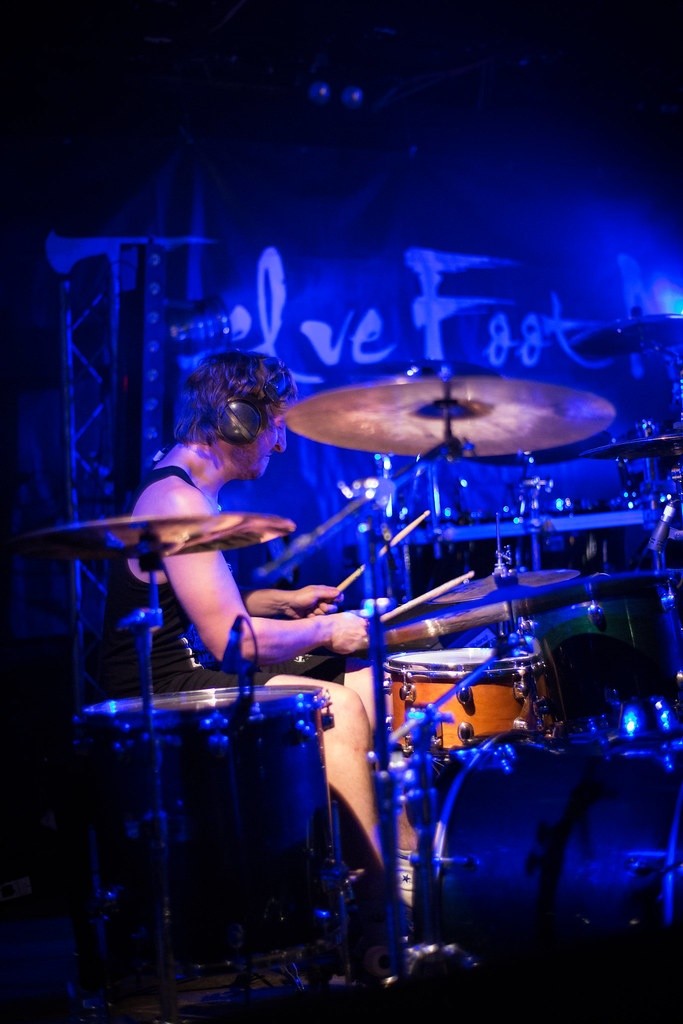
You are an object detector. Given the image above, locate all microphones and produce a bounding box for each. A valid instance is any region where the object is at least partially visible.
[637,503,676,569]
[221,620,244,674]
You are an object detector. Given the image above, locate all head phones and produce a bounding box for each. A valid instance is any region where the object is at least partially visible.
[214,359,291,445]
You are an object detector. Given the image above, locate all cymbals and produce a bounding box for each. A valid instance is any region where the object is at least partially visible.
[16,514,297,558]
[567,313,683,359]
[580,434,682,461]
[425,565,581,606]
[284,374,619,458]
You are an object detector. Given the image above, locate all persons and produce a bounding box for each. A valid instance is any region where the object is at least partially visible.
[100,350,418,906]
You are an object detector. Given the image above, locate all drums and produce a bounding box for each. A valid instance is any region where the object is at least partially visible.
[72,682,346,973]
[400,725,683,1024]
[382,647,555,748]
